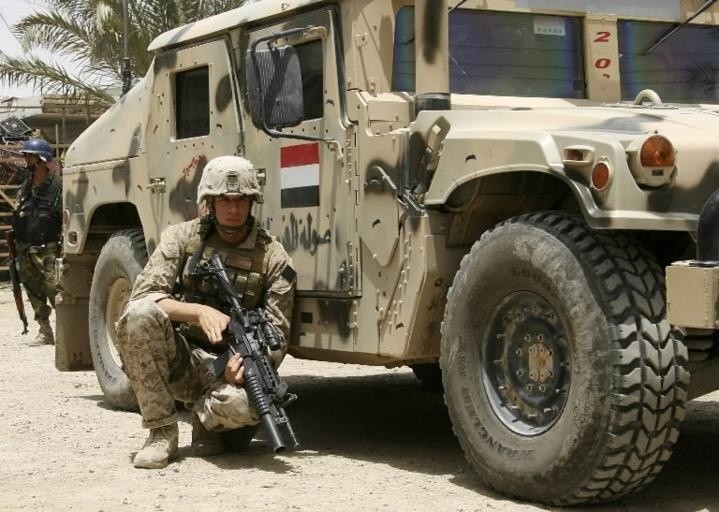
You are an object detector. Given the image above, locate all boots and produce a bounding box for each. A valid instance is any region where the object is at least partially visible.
[29,318,55,346]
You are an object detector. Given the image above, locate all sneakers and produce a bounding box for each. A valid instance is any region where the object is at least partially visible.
[191,406,224,456]
[133,421,180,468]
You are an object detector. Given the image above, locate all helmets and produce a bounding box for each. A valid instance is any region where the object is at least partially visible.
[19,139,54,162]
[196,156,264,205]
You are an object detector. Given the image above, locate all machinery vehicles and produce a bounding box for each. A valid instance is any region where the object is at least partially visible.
[53,2,718,508]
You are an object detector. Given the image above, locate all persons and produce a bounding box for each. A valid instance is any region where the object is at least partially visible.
[8,138,64,346]
[113,155,297,470]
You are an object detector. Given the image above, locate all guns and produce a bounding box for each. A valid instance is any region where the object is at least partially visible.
[5,229,29,335]
[184,254,300,453]
